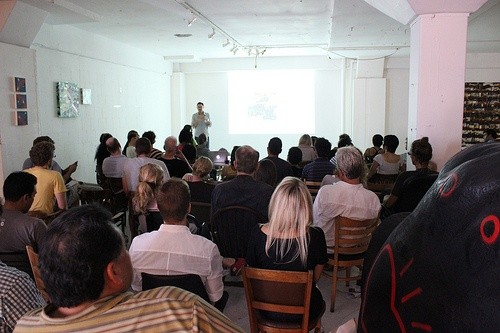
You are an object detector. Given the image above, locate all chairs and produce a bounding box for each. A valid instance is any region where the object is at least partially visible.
[25,181,397,333]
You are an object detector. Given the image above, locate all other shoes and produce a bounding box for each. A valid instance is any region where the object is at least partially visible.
[354,285,361,292]
[214,291,228,313]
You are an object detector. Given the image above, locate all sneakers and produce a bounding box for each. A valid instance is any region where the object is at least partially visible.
[230,258,247,277]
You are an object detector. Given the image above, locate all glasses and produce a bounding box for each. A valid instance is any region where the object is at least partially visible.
[407,151,416,155]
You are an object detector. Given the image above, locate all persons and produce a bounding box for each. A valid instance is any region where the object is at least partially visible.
[191,101,211,148]
[94,124,217,225]
[0,171,48,275]
[364,134,383,163]
[12,206,246,333]
[0,262,46,333]
[210,145,274,225]
[313,147,381,296]
[482,129,497,142]
[23,142,69,215]
[337,143,500,333]
[256,134,317,187]
[329,134,362,165]
[381,139,438,212]
[182,156,220,203]
[22,135,76,185]
[132,162,245,275]
[245,176,328,325]
[302,138,337,183]
[370,134,407,172]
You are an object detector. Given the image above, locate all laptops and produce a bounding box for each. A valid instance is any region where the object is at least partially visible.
[207,151,228,165]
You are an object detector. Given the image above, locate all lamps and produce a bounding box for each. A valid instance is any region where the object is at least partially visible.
[188,17,238,55]
